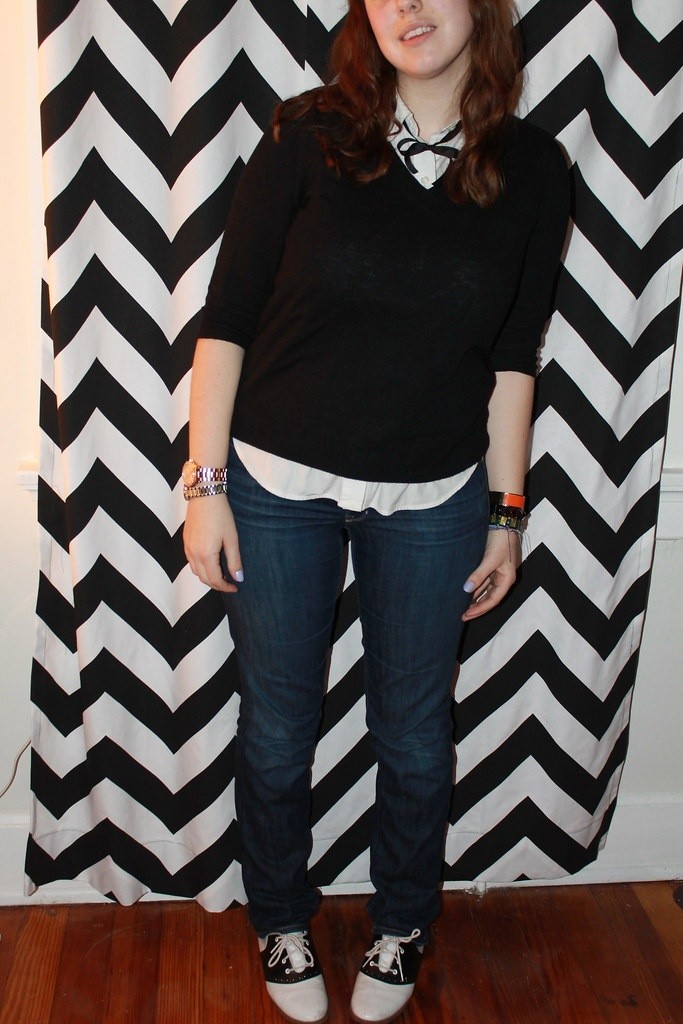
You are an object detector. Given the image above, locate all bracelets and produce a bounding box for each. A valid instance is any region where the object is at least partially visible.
[184,484,228,502]
[488,489,526,563]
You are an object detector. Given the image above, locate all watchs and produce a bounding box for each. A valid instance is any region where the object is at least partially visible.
[181,460,229,487]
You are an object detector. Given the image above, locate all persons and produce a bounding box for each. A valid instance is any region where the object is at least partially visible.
[182,0,574,1024]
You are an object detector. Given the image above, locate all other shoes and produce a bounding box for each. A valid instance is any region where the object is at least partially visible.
[351,918,433,1020]
[259,922,328,1022]
